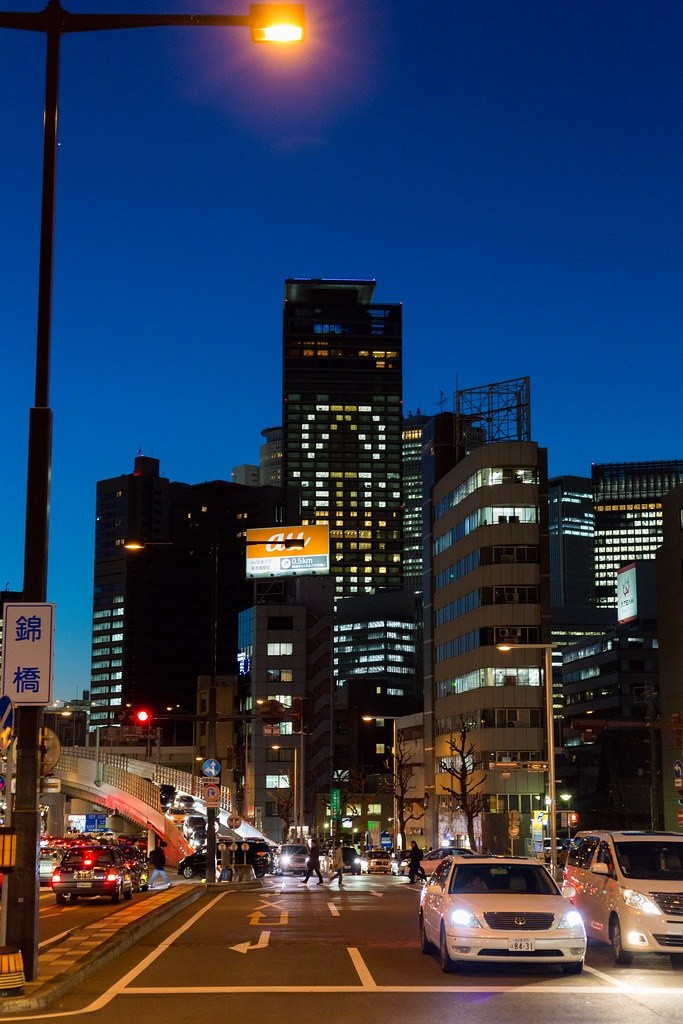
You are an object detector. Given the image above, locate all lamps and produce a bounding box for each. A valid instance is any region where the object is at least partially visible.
[311,571,316,575]
[270,574,274,577]
[249,574,255,578]
[293,572,297,576]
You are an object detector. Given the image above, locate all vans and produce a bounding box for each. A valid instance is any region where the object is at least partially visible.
[177,841,275,877]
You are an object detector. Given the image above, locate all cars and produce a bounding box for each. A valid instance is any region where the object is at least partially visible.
[404,847,480,881]
[274,844,309,875]
[305,840,361,875]
[40,831,148,903]
[418,854,587,974]
[361,850,411,875]
[543,837,574,859]
[561,830,683,966]
[143,777,264,850]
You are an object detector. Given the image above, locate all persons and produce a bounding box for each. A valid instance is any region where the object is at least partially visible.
[330,842,347,886]
[300,839,323,885]
[401,840,427,885]
[149,842,174,888]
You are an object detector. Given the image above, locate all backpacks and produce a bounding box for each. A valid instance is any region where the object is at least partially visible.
[417,849,423,861]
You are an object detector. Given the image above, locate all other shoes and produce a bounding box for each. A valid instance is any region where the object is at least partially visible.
[301,880,307,884]
[407,881,414,884]
[425,876,427,881]
[167,883,174,886]
[317,881,323,884]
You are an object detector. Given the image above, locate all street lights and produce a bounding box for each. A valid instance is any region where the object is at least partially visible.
[497,642,557,881]
[256,699,303,844]
[124,539,304,883]
[560,792,572,839]
[362,715,397,851]
[55,699,113,754]
[167,707,194,795]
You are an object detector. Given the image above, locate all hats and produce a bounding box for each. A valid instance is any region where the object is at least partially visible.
[160,842,167,846]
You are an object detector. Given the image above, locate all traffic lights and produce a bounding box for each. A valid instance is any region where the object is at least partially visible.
[570,814,577,823]
[114,708,150,721]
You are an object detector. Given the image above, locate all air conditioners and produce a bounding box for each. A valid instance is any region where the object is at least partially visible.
[510,628,522,637]
[499,629,509,636]
[505,592,518,604]
[505,720,518,728]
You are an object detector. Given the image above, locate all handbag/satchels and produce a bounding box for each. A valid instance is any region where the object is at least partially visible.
[150,850,155,858]
[307,861,309,866]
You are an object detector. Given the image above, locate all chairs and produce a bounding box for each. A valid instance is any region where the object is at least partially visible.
[509,876,527,891]
[72,856,82,862]
[666,854,682,870]
[98,855,110,862]
